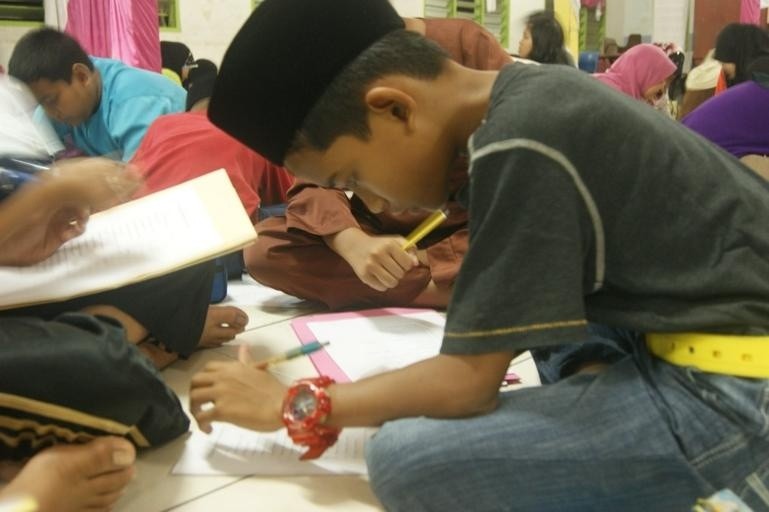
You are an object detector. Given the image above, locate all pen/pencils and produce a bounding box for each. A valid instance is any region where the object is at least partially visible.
[256,341,329,369]
[401,210,450,252]
[10,158,50,170]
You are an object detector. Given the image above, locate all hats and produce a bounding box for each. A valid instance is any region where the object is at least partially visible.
[206,0,408,170]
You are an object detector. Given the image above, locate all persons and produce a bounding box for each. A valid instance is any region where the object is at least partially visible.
[189,1,769,512]
[1,26,296,511]
[243,17,514,310]
[518,11,768,160]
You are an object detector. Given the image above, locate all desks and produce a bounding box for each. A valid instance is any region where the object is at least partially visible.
[107,271,543,512]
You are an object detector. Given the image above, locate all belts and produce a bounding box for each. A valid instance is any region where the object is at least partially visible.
[643,329,769,381]
